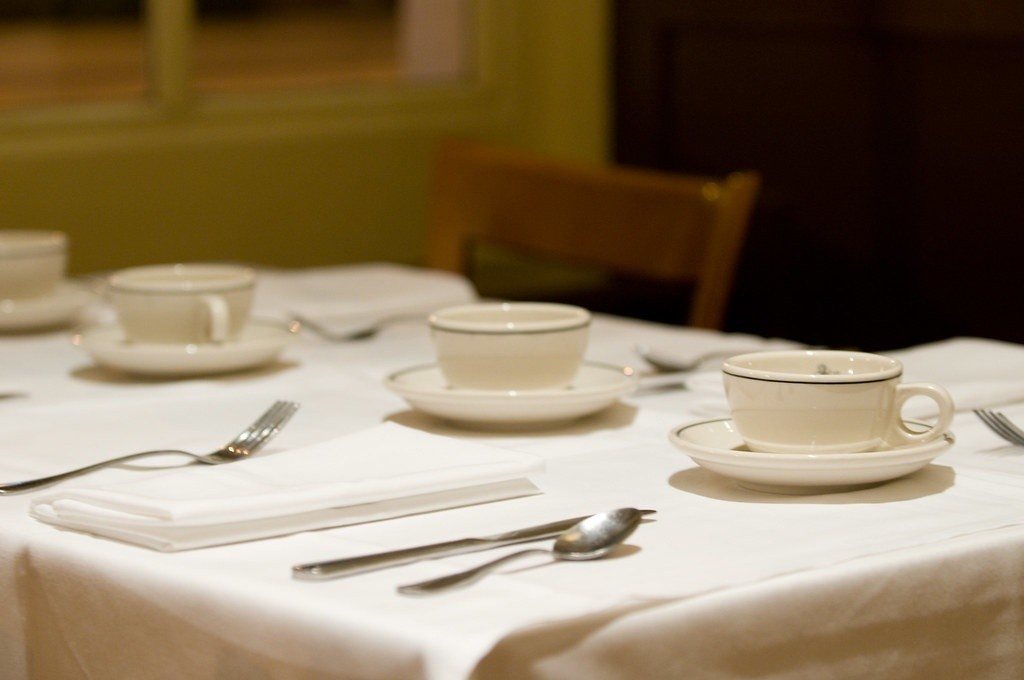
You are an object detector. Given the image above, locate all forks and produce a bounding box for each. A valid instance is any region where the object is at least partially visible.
[0,399,300,495]
[290,312,428,344]
[972,408,1024,448]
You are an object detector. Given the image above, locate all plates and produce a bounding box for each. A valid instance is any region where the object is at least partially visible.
[383,361,633,430]
[0,289,84,328]
[668,416,955,494]
[84,320,288,374]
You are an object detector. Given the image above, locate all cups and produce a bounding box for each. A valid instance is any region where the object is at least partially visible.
[722,350,953,453]
[1,230,68,298]
[108,264,253,345]
[428,301,591,390]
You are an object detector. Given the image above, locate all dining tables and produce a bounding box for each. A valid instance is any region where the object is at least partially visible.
[0,265,1023,680]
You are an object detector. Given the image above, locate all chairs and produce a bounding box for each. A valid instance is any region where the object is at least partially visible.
[430,133,759,330]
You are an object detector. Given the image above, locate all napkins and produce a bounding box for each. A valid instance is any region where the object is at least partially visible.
[885,339,1022,420]
[23,422,547,551]
[266,264,477,343]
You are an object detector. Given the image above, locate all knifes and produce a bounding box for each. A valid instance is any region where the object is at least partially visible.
[290,509,658,579]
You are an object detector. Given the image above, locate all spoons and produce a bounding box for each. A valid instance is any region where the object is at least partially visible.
[644,349,756,375]
[397,508,642,597]
